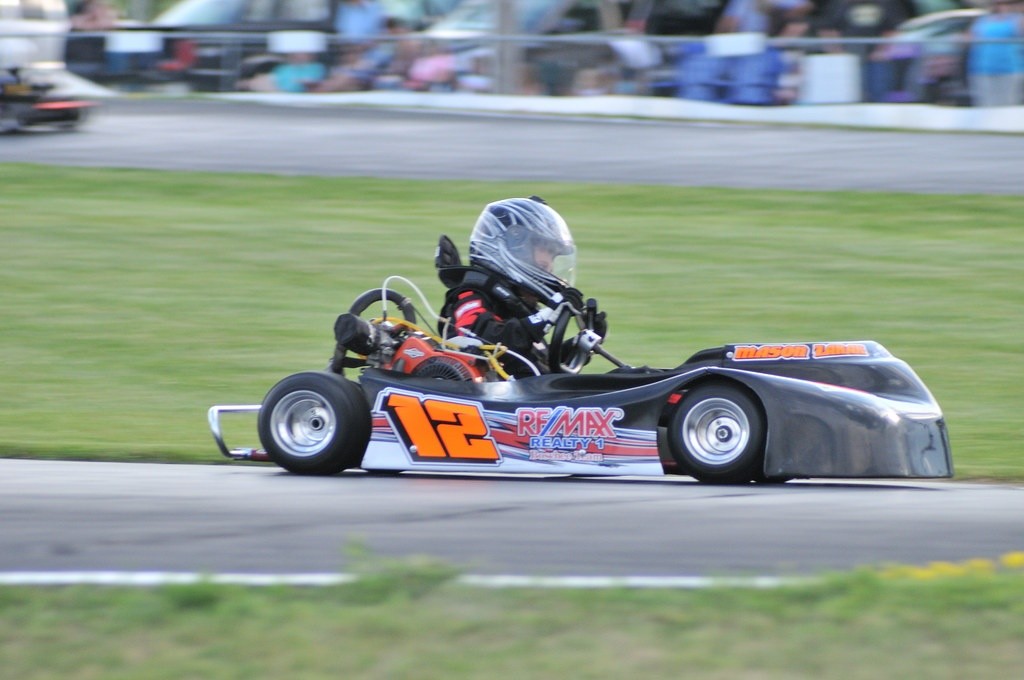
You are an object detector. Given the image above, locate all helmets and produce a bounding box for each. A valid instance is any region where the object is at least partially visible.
[464,195,575,310]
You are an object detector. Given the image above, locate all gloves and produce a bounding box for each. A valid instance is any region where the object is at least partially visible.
[520,288,583,343]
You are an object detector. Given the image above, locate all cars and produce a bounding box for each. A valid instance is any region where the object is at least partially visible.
[65,0,438,93]
[352,0,630,96]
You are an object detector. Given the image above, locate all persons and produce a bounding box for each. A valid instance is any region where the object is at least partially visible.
[438,197,606,378]
[12,0,1024,108]
